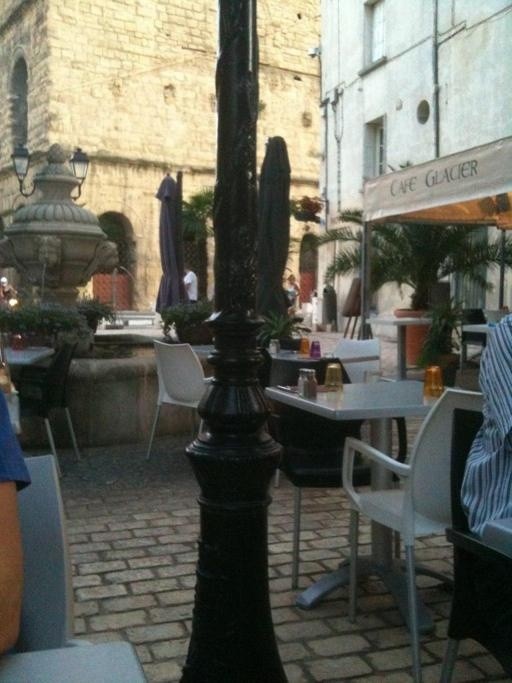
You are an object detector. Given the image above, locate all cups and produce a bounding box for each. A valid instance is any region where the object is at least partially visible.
[424,365,444,399]
[310,341,321,357]
[300,338,310,355]
[325,363,342,391]
[13,333,24,350]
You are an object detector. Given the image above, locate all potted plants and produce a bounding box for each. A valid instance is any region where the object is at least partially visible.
[314,195,512,384]
[76,292,116,337]
[256,308,308,354]
[162,299,213,345]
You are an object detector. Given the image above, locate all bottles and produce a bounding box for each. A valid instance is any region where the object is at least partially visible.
[298,368,317,400]
[269,338,279,353]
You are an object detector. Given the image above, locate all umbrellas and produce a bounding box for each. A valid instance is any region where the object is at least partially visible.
[154,173,188,339]
[254,135,292,321]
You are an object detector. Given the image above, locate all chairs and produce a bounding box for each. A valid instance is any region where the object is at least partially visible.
[147,337,215,461]
[10,338,84,477]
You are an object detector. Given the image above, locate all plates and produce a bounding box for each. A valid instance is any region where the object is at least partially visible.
[278,385,325,392]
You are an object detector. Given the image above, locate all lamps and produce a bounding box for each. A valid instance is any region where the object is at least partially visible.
[11,140,36,196]
[67,147,89,199]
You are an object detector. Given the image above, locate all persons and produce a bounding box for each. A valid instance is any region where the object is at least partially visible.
[0,382,32,655]
[459,311,511,540]
[1,276,18,305]
[180,264,199,304]
[281,274,301,317]
